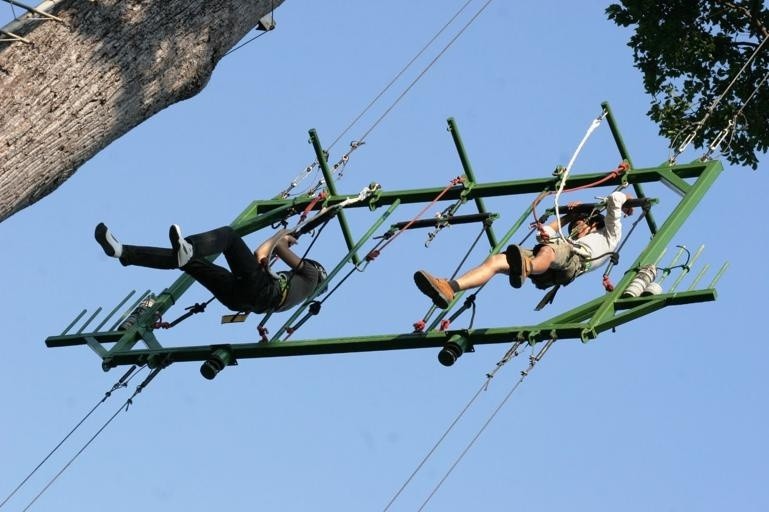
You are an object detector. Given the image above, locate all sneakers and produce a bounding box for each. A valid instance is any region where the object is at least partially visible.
[414,269,454,310]
[169,224,189,268]
[506,244,531,288]
[95,222,122,258]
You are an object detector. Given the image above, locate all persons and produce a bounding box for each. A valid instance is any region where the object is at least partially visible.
[412,191,634,309]
[94,223,329,313]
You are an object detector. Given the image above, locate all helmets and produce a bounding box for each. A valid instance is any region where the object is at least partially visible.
[303,258,328,297]
